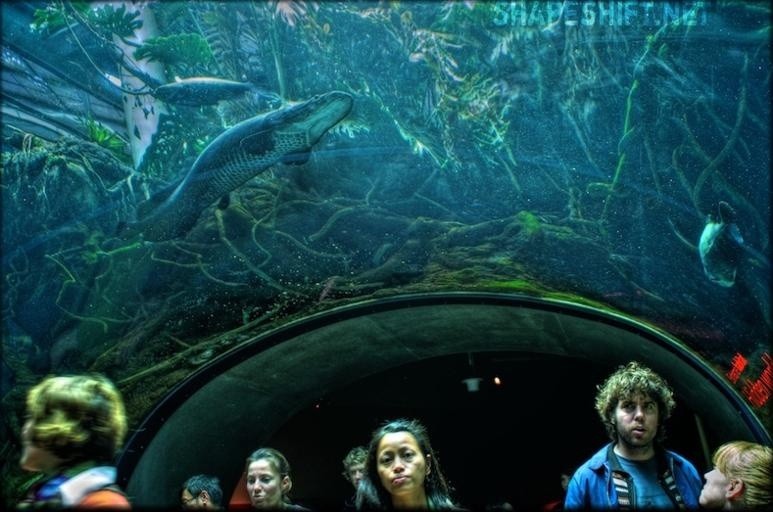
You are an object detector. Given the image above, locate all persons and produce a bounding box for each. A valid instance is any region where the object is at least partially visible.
[353,415,458,510]
[697,440,772,511]
[11,371,129,511]
[337,444,372,506]
[559,358,705,512]
[176,473,223,510]
[241,445,310,512]
[542,464,571,510]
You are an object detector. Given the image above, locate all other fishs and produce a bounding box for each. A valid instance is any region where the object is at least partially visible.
[116,91,353,279]
[698,201,769,287]
[150,76,255,107]
